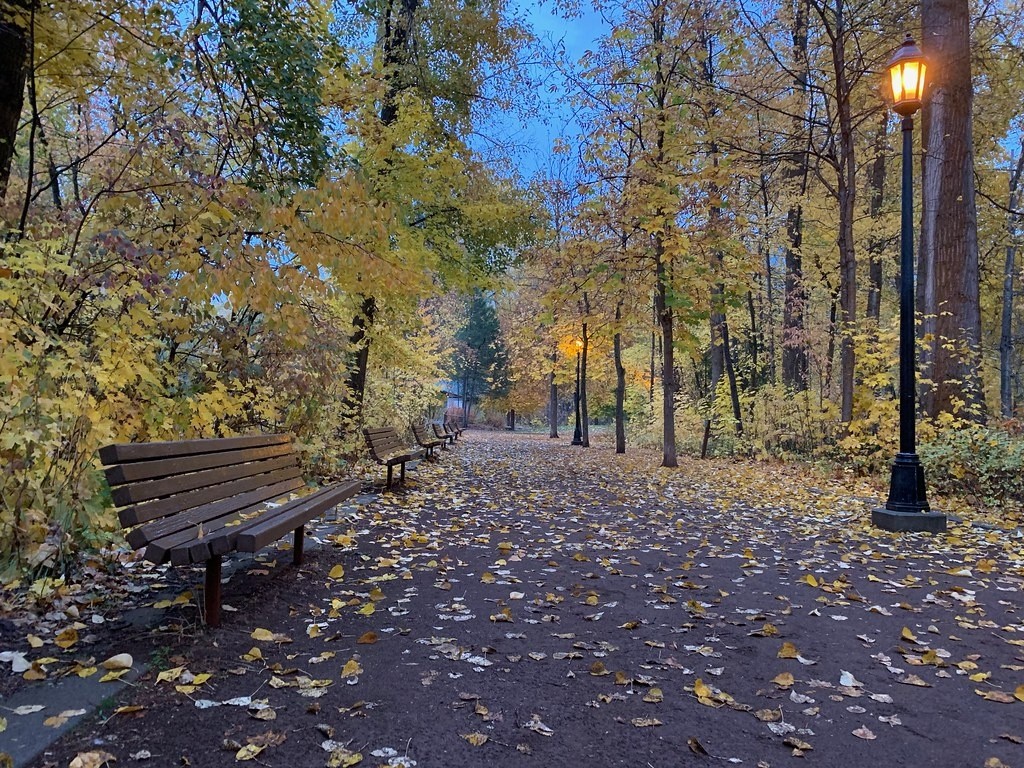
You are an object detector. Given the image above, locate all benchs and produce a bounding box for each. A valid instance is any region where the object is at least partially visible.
[363,426,425,490]
[432,421,466,450]
[412,425,443,459]
[99,433,360,630]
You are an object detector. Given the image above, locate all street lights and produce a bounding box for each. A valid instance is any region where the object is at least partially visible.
[885,31,938,513]
[573,336,587,442]
